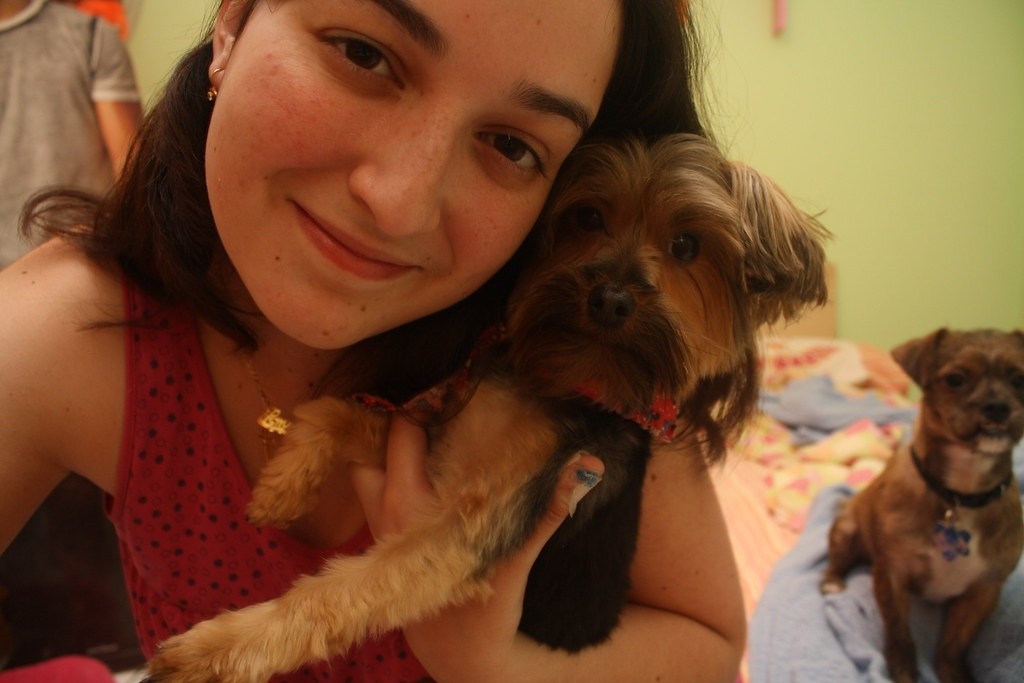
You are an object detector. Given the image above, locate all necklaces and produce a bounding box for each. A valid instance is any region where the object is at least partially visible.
[248,357,290,435]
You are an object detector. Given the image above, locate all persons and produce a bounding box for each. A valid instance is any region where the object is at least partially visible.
[0,0,145,268]
[0,0,747,683]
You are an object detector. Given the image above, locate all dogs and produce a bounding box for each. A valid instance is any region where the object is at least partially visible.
[818,326,1024,683]
[142,134,833,683]
